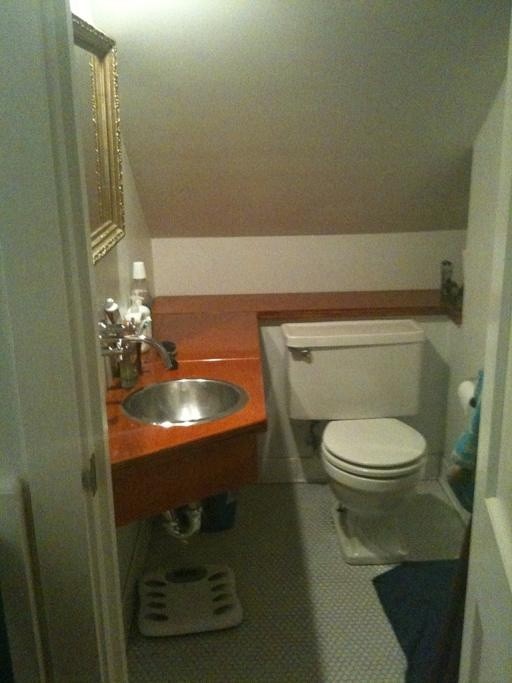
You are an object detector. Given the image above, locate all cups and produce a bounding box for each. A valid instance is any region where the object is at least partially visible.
[119,340,141,389]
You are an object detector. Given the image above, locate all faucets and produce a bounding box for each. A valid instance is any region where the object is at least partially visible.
[99,333,177,372]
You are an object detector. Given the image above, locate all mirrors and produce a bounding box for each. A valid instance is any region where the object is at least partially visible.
[69,8,125,266]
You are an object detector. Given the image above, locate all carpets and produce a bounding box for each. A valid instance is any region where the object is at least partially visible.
[372,554,465,683]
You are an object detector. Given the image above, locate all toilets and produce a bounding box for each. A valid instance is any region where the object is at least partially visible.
[280,319,428,566]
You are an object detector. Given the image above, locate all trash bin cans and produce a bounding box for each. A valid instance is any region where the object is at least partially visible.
[176,487,237,533]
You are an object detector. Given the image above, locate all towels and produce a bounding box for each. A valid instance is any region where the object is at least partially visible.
[445,370,484,512]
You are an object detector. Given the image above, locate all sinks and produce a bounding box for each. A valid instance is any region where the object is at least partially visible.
[120,376,249,428]
